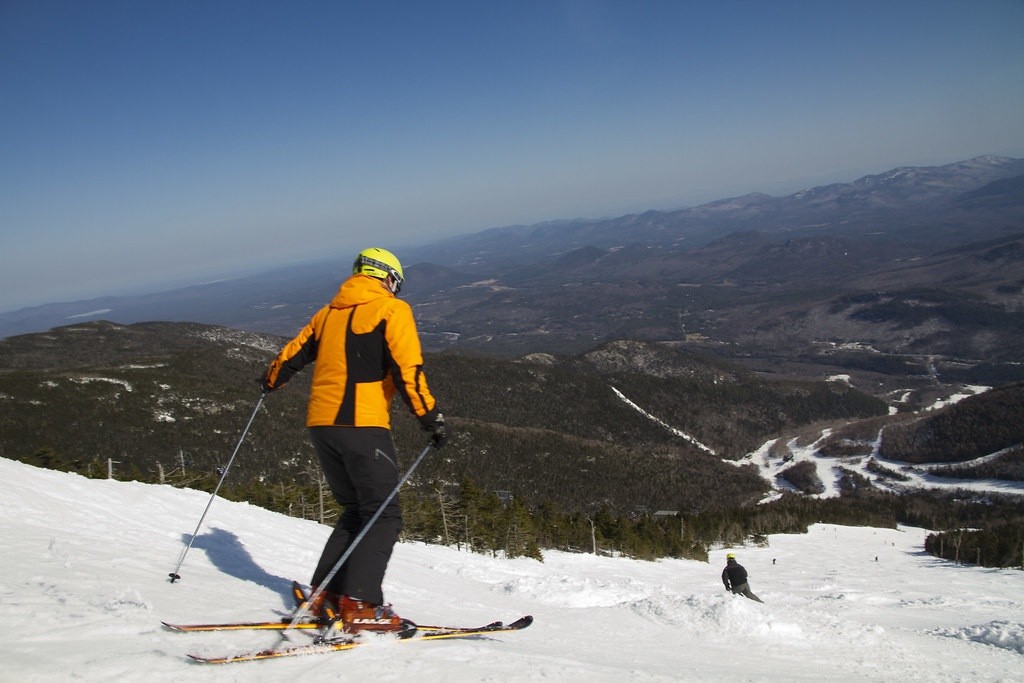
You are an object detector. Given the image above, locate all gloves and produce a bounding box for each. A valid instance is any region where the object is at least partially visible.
[419,413,448,449]
[260,375,275,393]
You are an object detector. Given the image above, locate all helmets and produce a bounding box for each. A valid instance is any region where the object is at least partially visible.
[352,247,406,282]
[727,553,735,559]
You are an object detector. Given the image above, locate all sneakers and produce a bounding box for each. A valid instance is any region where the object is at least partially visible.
[338,596,401,632]
[309,584,341,626]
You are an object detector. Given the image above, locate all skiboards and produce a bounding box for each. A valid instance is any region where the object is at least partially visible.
[160,615,534,664]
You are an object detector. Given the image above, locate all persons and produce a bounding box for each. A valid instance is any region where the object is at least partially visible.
[722,554,763,603]
[876,556,878,561]
[773,559,775,564]
[261,247,449,634]
